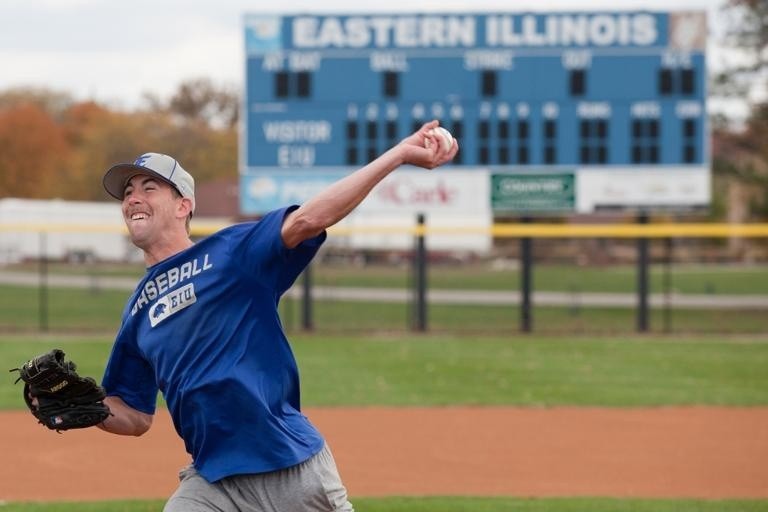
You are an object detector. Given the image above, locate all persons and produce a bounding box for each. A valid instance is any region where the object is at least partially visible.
[22,119,458,511]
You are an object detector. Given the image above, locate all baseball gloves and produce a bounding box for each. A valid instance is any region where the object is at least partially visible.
[19,350,109,430]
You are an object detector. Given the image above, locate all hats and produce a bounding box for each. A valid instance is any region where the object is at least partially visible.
[103,153,195,220]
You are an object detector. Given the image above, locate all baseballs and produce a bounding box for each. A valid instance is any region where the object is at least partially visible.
[425,129,453,153]
